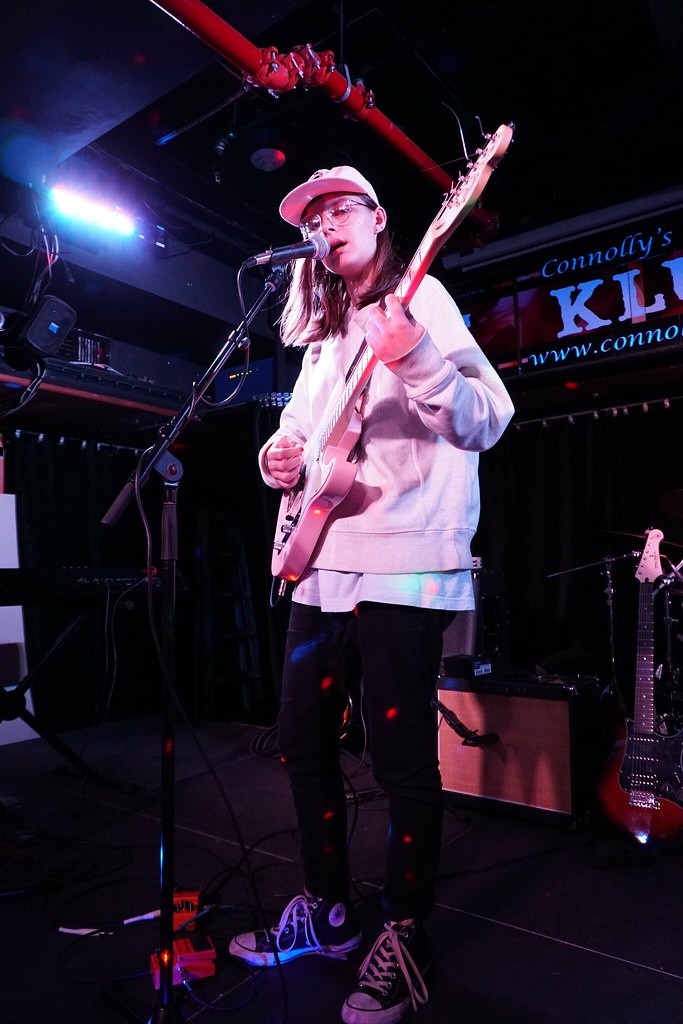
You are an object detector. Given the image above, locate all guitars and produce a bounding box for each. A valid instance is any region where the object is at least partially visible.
[269,122,517,582]
[593,526,683,856]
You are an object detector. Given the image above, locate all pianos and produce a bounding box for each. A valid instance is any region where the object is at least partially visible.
[0,565,161,608]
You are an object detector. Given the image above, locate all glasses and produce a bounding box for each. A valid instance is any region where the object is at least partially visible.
[298,198,374,240]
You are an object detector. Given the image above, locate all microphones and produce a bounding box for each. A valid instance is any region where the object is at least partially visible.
[462,733,498,746]
[242,233,331,269]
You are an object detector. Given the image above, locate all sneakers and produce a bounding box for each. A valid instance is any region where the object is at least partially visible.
[342,916,431,1023]
[230,890,362,967]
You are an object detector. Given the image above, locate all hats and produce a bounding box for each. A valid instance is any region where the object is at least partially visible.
[278,165,380,228]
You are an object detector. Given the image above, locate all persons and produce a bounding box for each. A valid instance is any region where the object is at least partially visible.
[228,165,516,1022]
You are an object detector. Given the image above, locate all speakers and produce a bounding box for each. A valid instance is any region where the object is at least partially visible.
[18,295,77,355]
[432,653,617,832]
